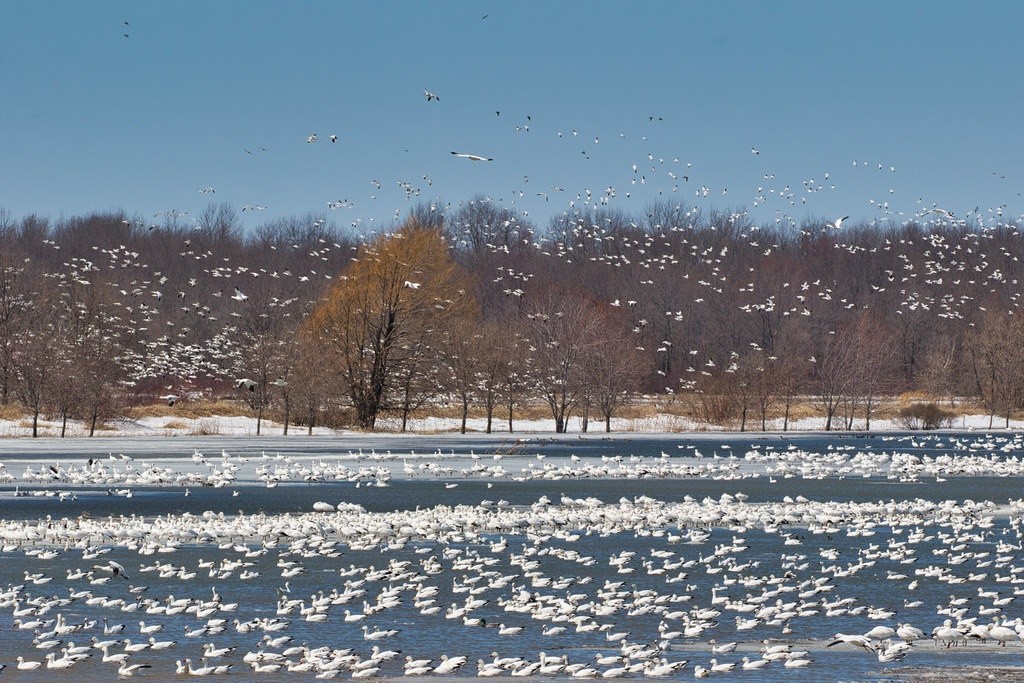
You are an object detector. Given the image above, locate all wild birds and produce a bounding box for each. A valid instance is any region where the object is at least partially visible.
[124,34,128,38]
[0,110,1024,681]
[481,14,489,19]
[424,87,440,102]
[124,22,128,26]
